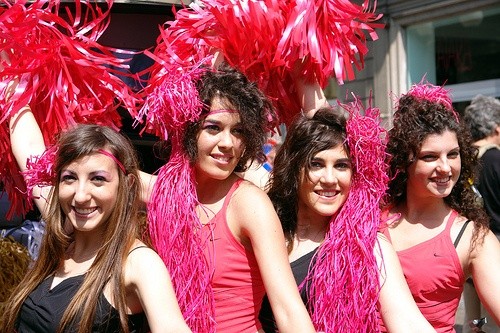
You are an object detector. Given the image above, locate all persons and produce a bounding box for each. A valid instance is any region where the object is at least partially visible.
[0,45,195,333]
[295,73,500,333]
[214,50,438,333]
[136,62,316,333]
[456,94,500,240]
